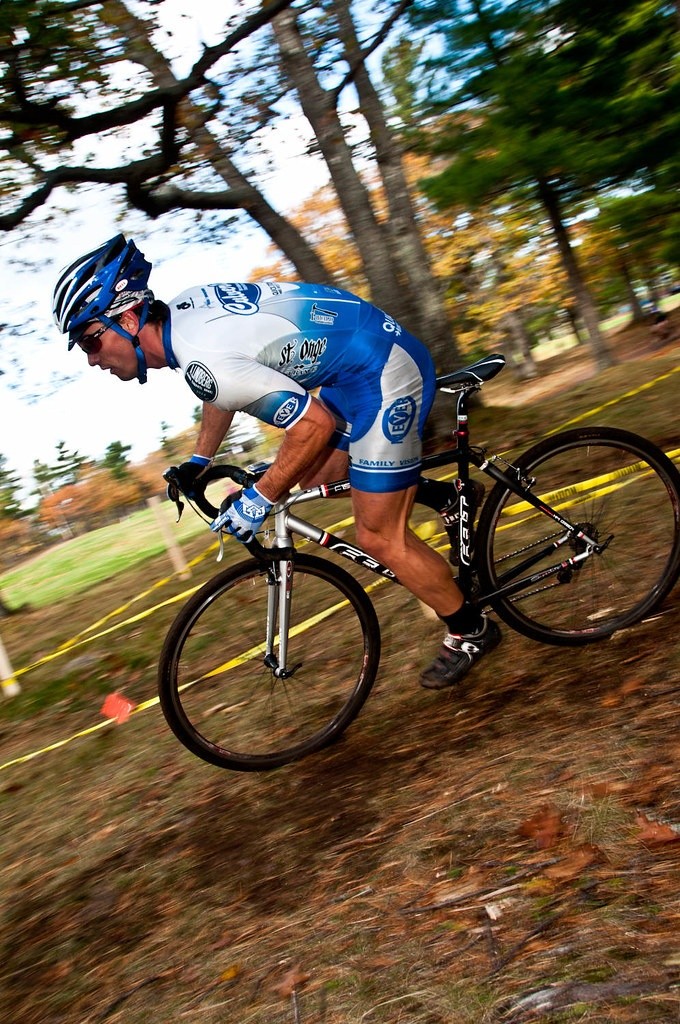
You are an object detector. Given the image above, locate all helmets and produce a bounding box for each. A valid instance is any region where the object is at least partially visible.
[52,234,152,334]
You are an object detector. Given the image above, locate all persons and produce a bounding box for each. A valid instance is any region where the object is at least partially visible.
[50,233,503,686]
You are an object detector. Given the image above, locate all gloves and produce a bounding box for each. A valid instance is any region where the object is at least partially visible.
[167,453,216,502]
[210,484,278,544]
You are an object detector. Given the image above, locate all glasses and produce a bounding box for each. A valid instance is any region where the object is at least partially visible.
[76,314,123,354]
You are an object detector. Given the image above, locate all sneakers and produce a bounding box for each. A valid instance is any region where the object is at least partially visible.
[420,614,501,690]
[438,477,486,568]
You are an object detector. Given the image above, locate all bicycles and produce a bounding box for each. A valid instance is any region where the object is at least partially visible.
[159,354,680,770]
